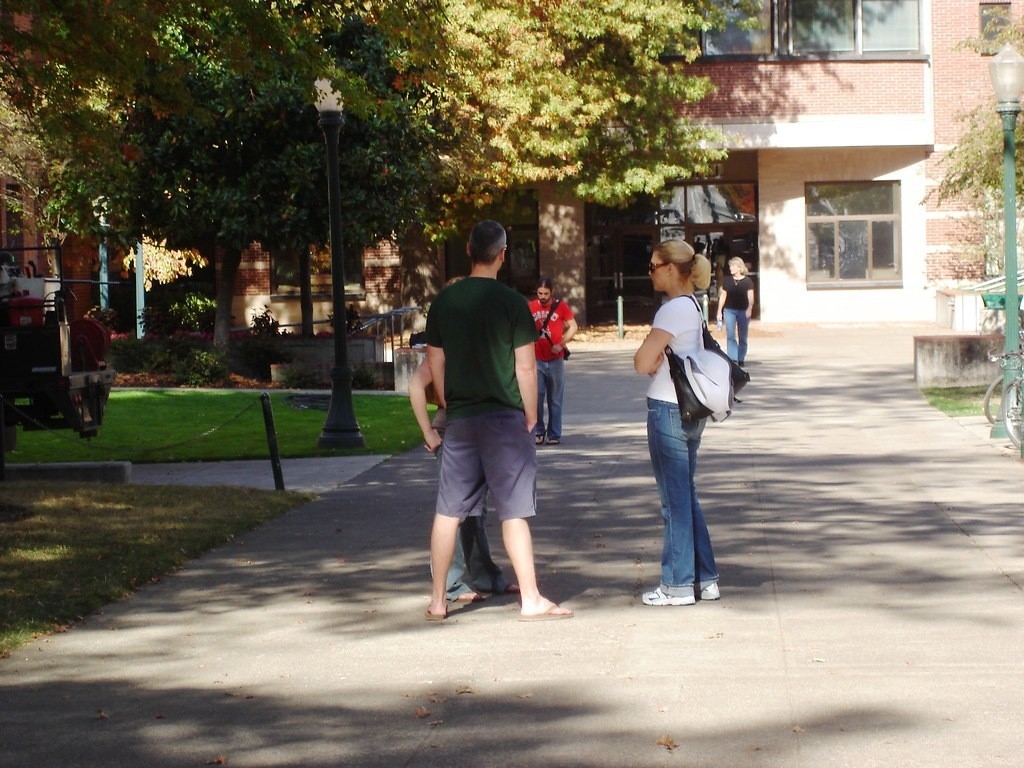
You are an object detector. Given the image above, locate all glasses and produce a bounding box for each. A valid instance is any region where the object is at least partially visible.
[648,261,672,273]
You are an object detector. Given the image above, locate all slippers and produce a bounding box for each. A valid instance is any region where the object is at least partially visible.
[518,604,574,621]
[456,592,486,602]
[423,604,444,619]
[504,584,520,593]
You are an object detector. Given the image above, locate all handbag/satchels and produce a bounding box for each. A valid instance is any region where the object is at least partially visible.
[665,345,734,423]
[564,347,570,361]
[679,294,750,403]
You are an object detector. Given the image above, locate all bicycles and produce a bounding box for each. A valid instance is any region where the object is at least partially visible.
[984,330,1024,426]
[986,347,1024,451]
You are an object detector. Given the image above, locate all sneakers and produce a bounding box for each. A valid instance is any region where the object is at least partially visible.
[694,582,720,599]
[642,583,695,605]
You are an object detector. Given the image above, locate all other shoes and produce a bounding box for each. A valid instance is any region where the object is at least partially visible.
[738,361,744,367]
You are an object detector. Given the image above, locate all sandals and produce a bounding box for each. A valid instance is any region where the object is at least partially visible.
[535,429,546,444]
[546,438,560,445]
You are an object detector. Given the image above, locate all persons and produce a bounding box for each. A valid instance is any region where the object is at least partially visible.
[633,240,722,605]
[425,220,575,621]
[716,257,755,368]
[526,275,578,447]
[407,276,522,601]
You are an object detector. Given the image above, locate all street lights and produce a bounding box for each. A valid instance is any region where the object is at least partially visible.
[310,76,366,451]
[986,43,1024,440]
